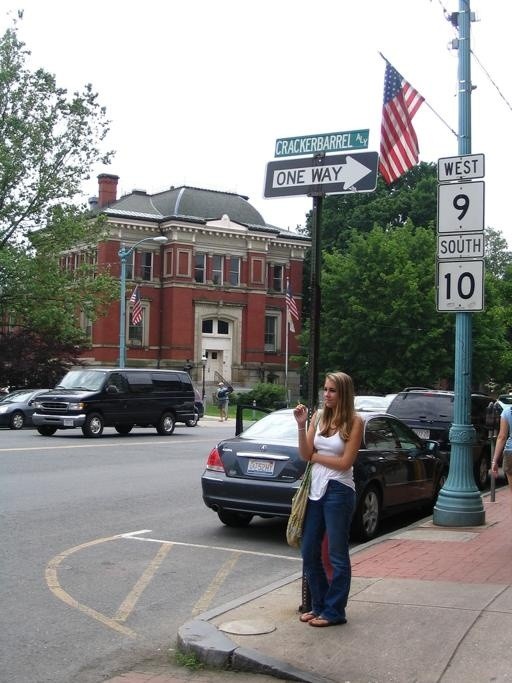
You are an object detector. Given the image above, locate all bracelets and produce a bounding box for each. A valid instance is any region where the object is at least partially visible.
[298,427,305,432]
[492,462,497,464]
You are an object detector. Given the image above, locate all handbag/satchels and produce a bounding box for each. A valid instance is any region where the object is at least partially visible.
[286,475,311,548]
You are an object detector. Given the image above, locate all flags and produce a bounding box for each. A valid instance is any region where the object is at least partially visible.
[380,61,427,187]
[130,286,143,326]
[284,279,299,322]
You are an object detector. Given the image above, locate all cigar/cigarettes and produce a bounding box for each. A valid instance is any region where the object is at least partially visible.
[297,400,304,412]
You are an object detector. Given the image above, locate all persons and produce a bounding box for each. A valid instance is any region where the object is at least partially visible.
[490,404,511,491]
[214,382,231,423]
[292,370,365,628]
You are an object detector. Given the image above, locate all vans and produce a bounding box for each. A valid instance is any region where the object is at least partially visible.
[31,366,195,436]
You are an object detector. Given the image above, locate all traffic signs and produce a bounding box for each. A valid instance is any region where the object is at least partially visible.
[262,127,381,201]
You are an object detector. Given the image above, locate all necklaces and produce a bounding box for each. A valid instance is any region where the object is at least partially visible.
[329,424,337,431]
[327,427,335,438]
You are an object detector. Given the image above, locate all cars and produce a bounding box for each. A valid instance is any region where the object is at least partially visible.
[0,389,54,429]
[201,407,453,540]
[387,385,511,489]
[186,388,204,427]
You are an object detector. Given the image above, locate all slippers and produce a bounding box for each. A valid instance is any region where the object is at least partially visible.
[300,610,318,622]
[309,614,347,627]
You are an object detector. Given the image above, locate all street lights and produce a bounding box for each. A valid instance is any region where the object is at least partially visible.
[117,236,168,369]
